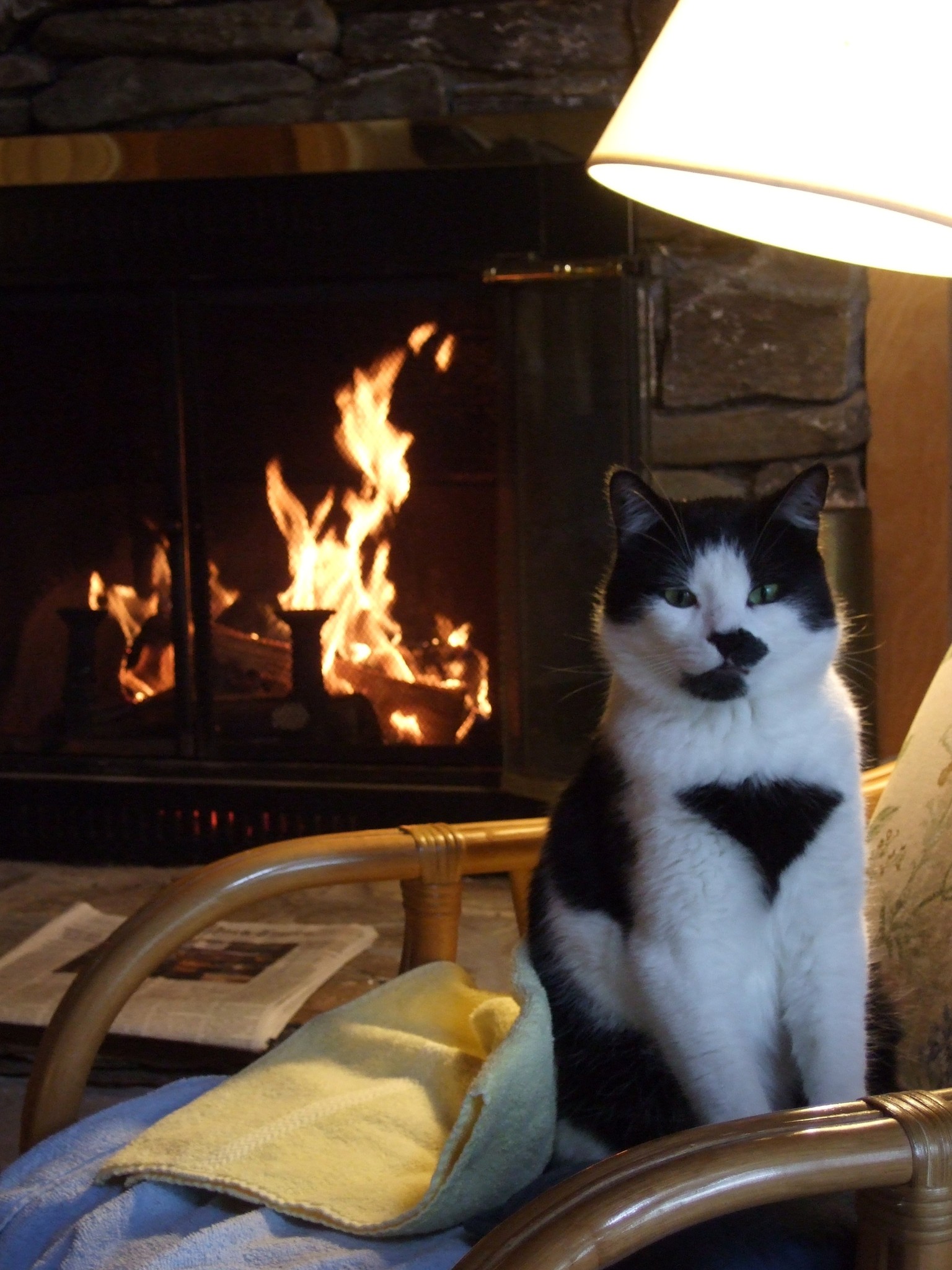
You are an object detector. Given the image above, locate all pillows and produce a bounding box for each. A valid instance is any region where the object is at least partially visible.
[867,643,952,1094]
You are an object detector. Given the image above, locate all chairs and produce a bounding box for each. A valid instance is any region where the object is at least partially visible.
[18,642,952,1270]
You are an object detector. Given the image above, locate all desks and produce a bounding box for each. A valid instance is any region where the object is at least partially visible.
[0,860,520,1086]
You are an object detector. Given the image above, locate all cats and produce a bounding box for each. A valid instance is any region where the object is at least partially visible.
[523,462,909,1163]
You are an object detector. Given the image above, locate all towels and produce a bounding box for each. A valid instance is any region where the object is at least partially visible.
[90,925,558,1243]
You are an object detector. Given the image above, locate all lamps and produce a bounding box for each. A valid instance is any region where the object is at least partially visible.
[584,0,952,279]
